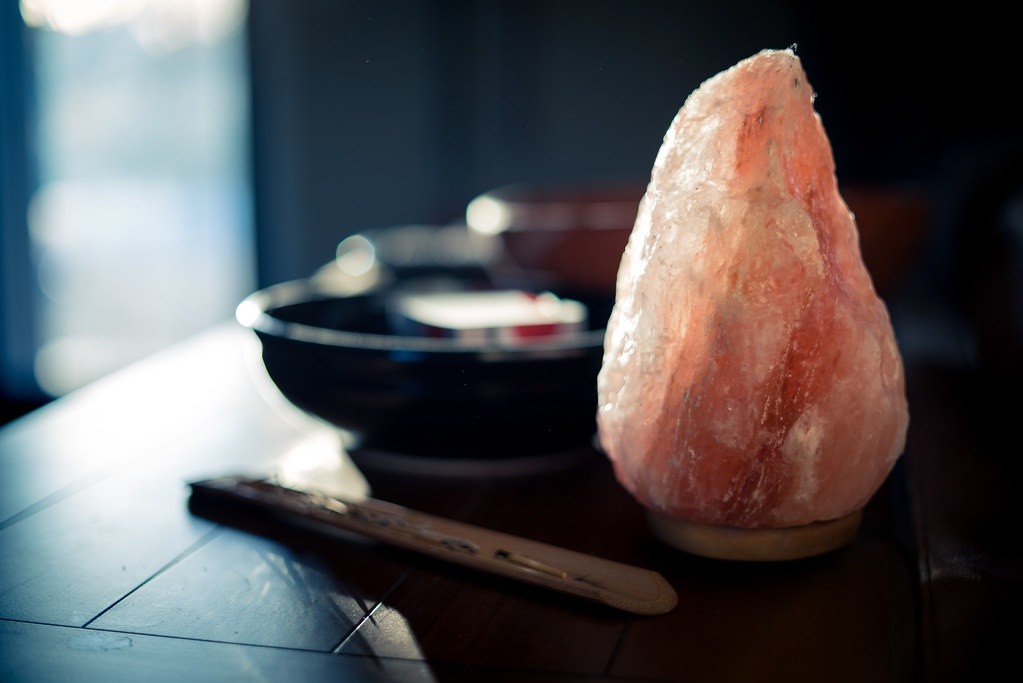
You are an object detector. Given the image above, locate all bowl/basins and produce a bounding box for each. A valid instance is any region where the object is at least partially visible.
[236,269,621,479]
[465,177,655,309]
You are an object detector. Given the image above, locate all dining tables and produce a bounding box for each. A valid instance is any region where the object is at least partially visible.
[0,312,1023,683]
